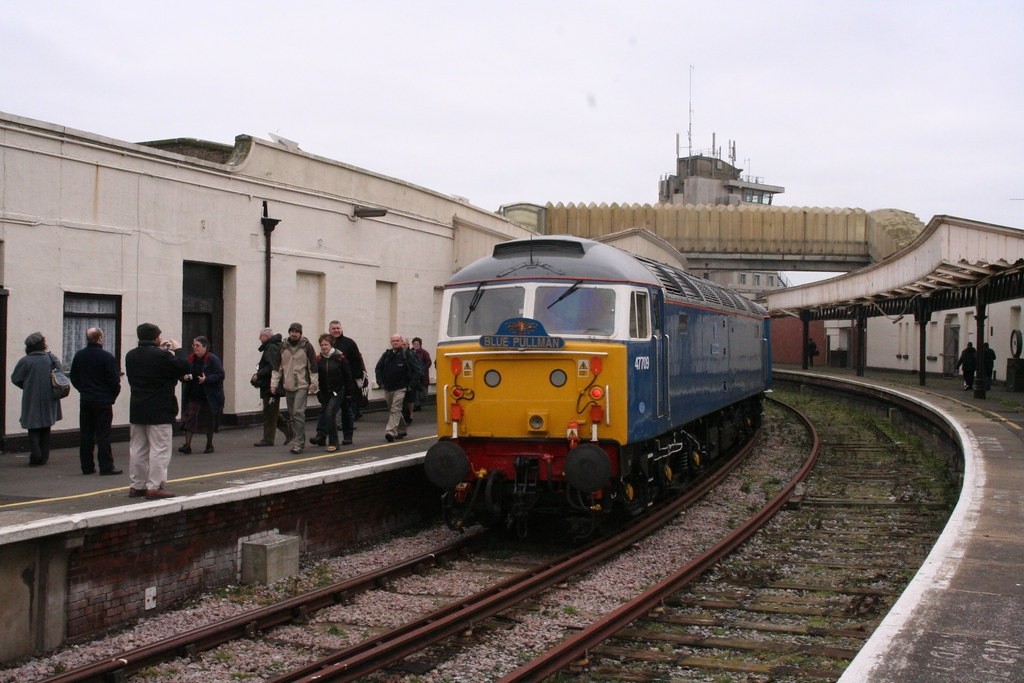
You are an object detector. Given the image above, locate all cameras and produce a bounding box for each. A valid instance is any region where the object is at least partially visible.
[165,343,172,349]
[187,374,192,380]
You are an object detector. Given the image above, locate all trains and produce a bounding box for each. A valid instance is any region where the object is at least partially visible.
[425,233,772,550]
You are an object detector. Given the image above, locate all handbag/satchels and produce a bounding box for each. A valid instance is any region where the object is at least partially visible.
[47,351,69,399]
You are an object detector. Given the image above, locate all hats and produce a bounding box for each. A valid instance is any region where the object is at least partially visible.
[137,324,161,341]
[289,323,302,333]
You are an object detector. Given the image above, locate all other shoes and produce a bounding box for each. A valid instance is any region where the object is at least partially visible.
[326,443,337,451]
[83,469,97,475]
[310,432,327,446]
[283,431,296,445]
[145,487,176,500]
[385,433,395,444]
[129,487,148,498]
[178,444,191,455]
[291,444,302,455]
[204,444,215,454]
[100,466,123,475]
[964,385,969,391]
[342,438,352,445]
[255,439,274,446]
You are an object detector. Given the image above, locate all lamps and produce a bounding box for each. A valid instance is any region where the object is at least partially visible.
[353,204,386,218]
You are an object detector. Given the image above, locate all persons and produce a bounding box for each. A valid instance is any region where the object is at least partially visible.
[177,336,226,455]
[955,342,978,390]
[125,322,189,500]
[375,334,424,440]
[250,327,295,447]
[977,340,997,390]
[69,326,121,474]
[11,330,71,465]
[807,337,818,368]
[410,337,432,412]
[329,321,368,430]
[271,323,352,454]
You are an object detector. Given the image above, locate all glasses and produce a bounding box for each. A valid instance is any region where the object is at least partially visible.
[192,343,205,348]
